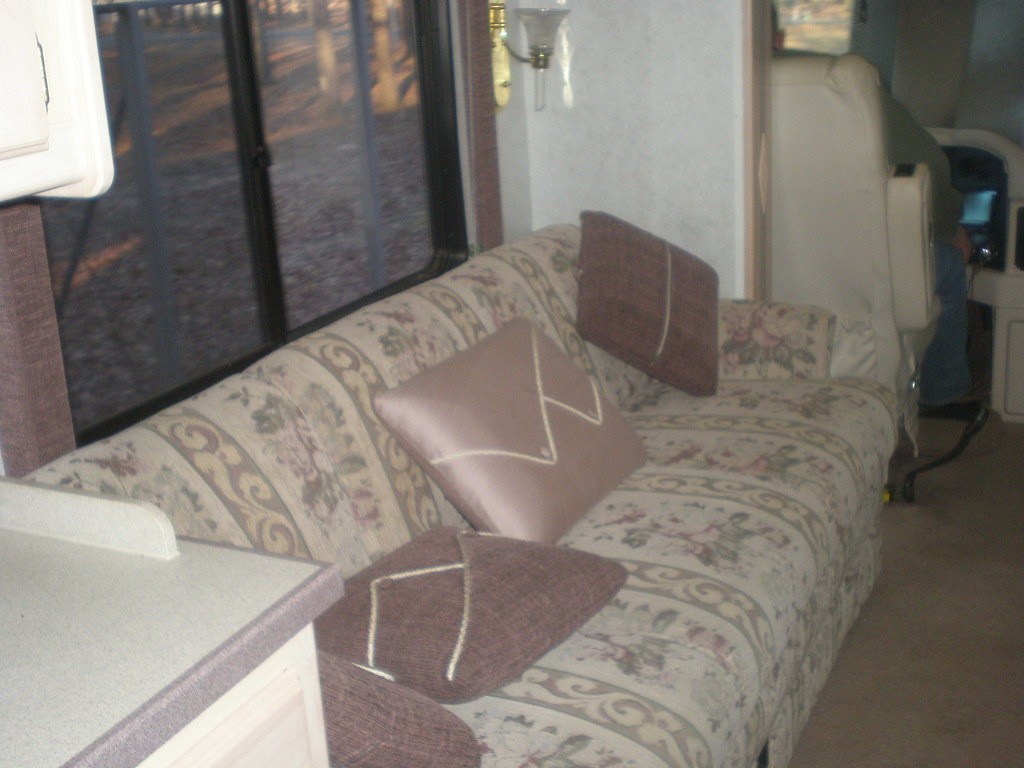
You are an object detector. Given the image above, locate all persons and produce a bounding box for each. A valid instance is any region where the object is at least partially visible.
[771,0,971,408]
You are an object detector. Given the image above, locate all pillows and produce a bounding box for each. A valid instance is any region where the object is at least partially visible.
[576,210,719,398]
[315,651,482,768]
[371,317,648,546]
[312,524,630,705]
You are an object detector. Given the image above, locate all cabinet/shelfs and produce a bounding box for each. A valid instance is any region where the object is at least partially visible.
[0,0,115,205]
[135,621,331,768]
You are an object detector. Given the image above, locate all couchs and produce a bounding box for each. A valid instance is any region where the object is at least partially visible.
[6,222,901,768]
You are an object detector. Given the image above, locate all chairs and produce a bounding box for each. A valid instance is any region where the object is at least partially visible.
[768,52,937,477]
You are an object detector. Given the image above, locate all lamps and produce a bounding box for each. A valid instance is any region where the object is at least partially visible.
[487,0,572,110]
